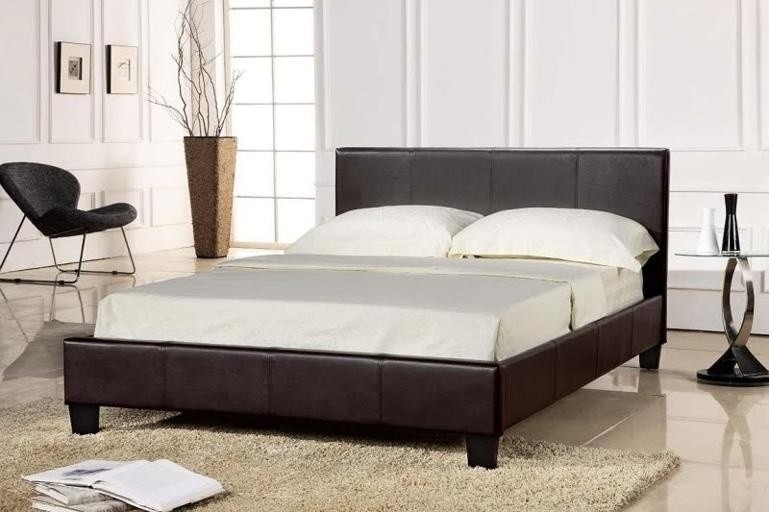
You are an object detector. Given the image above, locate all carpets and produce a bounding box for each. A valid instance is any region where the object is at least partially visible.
[0,396,679,512]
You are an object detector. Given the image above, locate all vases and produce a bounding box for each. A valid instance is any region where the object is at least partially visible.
[183,137,236,258]
[106,45,137,94]
[698,207,719,255]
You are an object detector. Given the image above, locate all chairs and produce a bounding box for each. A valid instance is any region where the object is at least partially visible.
[0,162,137,285]
[0,282,94,382]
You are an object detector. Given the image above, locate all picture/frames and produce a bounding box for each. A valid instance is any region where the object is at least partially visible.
[56,42,91,94]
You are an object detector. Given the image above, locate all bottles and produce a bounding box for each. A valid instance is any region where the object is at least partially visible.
[695,207,719,254]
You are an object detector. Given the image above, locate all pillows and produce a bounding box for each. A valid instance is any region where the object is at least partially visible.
[448,207,659,273]
[283,204,483,259]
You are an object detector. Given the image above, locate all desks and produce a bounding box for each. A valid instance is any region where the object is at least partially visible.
[674,251,769,387]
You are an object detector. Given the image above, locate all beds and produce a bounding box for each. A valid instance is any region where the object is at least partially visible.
[63,147,670,469]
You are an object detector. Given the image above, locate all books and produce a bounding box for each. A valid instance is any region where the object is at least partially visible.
[20,457,226,511]
[31,483,119,506]
[29,496,134,512]
